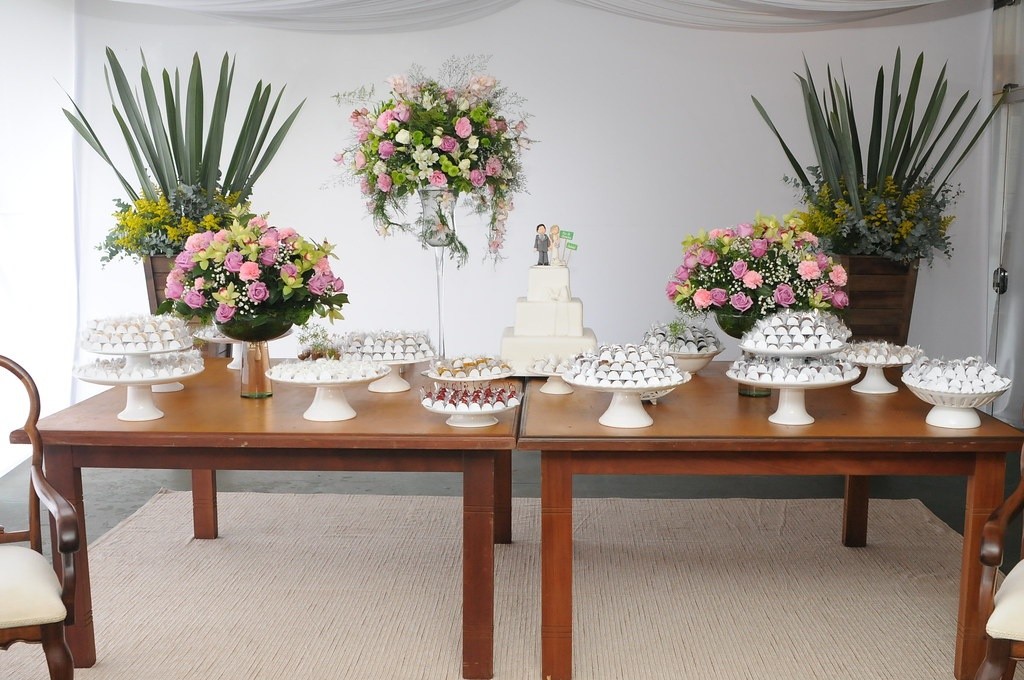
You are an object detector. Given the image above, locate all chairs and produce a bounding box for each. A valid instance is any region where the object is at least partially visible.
[974,442,1024,680]
[0,354,79,680]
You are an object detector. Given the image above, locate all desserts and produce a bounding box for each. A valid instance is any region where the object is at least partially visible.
[727,307,1012,394]
[76,315,204,380]
[268,322,719,413]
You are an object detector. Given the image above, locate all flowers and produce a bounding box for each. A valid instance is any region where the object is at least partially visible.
[155,203,351,342]
[53,45,307,269]
[333,54,541,270]
[751,45,1010,270]
[670,211,849,336]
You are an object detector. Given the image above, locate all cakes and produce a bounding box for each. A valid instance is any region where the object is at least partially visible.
[501,265,597,365]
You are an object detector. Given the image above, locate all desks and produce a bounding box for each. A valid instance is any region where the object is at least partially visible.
[517,361,1024,680]
[9,359,523,680]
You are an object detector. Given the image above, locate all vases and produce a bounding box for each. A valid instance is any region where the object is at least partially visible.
[716,315,774,396]
[836,256,920,371]
[211,312,293,399]
[143,254,232,359]
[418,188,458,377]
